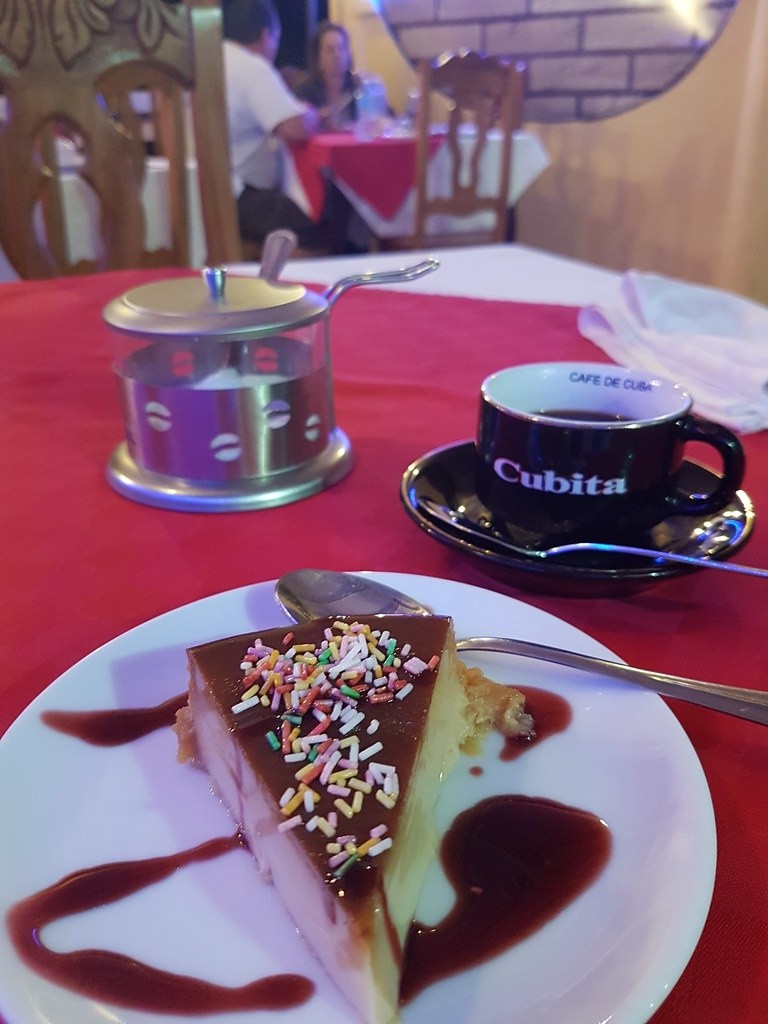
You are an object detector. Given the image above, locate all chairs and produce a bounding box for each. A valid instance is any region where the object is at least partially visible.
[412,48,530,252]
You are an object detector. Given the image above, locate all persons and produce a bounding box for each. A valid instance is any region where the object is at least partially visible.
[278,20,377,255]
[220,1,335,250]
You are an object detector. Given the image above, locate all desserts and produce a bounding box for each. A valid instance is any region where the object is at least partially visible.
[175,611,526,1024]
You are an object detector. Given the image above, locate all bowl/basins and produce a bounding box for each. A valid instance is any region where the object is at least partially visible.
[100,260,440,511]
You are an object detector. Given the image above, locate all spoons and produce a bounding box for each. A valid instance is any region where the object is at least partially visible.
[274,569,768,728]
[416,497,767,577]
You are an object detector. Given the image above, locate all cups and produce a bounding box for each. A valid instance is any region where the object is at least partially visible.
[475,361,745,548]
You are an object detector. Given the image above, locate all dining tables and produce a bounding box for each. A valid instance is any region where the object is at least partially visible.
[0,241,768,1024]
[60,156,252,281]
[296,124,551,256]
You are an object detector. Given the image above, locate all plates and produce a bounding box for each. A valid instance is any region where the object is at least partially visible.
[399,438,755,592]
[0,570,718,1023]
